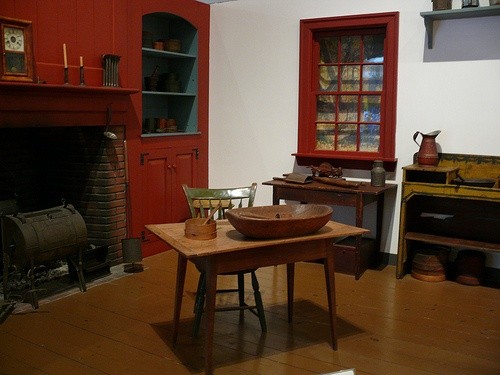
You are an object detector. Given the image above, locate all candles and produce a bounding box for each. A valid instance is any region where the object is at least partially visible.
[63,44,67,68]
[80,56,83,67]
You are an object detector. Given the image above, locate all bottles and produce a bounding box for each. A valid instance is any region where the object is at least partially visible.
[371,160,385,188]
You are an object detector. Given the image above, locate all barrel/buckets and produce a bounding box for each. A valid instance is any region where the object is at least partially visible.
[454,249,487,285]
[410,242,449,281]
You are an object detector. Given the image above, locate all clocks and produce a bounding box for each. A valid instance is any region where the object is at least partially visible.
[0,16,35,83]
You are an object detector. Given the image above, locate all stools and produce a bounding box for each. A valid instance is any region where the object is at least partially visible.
[192,200,231,220]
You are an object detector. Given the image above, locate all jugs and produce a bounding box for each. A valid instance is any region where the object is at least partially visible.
[413,129,441,168]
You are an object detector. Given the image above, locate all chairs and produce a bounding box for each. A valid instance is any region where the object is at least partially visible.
[181,183,267,335]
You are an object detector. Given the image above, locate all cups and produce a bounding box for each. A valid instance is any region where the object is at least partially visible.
[141,36,182,134]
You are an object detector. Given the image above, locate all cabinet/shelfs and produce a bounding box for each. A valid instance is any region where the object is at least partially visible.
[141,47,198,137]
[395,153,500,279]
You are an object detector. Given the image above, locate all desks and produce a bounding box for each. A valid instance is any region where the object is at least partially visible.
[143,217,371,375]
[262,175,399,280]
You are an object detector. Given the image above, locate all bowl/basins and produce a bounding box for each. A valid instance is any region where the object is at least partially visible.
[452,178,495,187]
[225,204,334,239]
